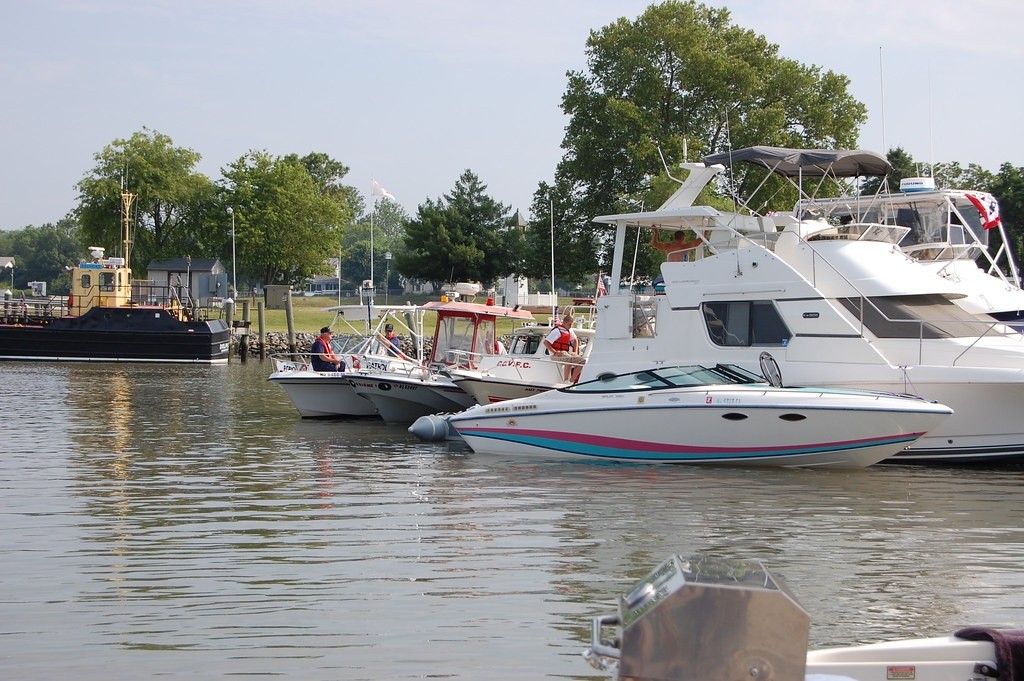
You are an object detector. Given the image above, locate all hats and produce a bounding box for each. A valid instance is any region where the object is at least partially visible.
[320,327,334,334]
[385,324,394,331]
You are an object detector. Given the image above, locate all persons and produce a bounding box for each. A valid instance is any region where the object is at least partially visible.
[543,315,586,385]
[651,224,706,262]
[377,324,400,358]
[311,327,345,372]
[480,331,507,357]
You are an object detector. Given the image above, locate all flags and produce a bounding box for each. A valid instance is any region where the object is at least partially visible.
[598,277,605,296]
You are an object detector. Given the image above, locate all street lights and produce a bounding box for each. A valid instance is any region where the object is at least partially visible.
[227,207,237,315]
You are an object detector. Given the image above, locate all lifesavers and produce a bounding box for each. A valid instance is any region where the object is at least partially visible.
[485,339,500,353]
[69,293,74,307]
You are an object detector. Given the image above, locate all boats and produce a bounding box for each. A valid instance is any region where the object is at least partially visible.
[443,351,955,469]
[265,47,1024,464]
[0,158,232,364]
